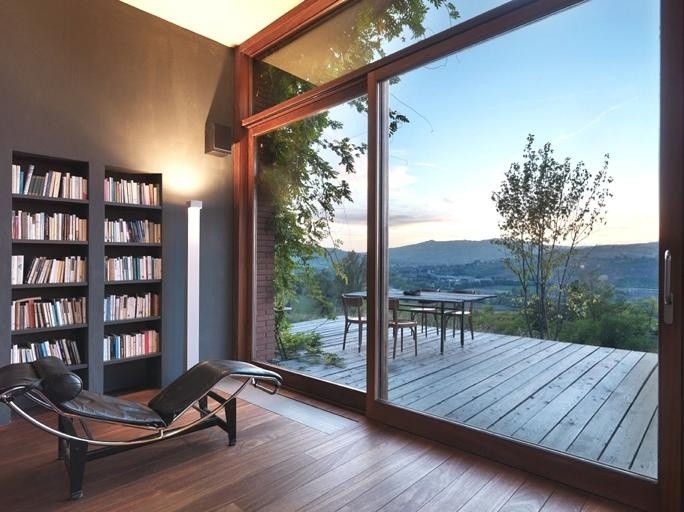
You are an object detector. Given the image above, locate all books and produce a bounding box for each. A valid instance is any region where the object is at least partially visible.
[8,161,160,368]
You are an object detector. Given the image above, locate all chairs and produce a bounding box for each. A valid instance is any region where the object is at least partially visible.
[0,355,283,500]
[9,146,164,420]
[341,286,499,361]
